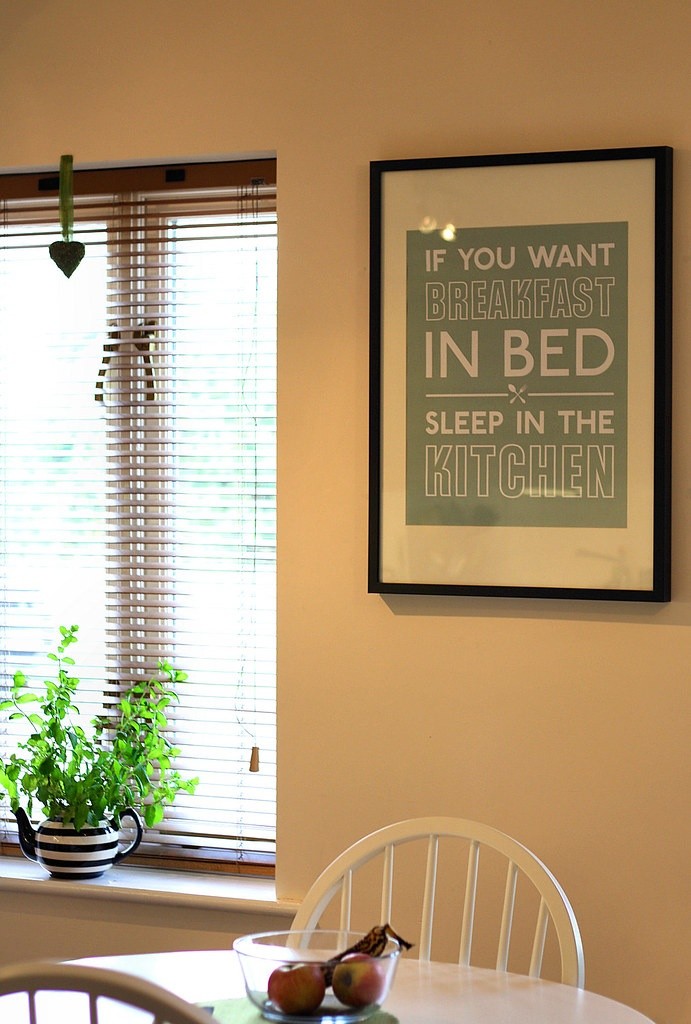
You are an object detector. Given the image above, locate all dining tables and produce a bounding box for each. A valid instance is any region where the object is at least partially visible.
[0,946,659,1024]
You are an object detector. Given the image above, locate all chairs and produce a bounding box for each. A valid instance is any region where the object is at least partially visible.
[284,814,590,992]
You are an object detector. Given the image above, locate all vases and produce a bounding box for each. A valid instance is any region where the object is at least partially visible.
[9,801,147,880]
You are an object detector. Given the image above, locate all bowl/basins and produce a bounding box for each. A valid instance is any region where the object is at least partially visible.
[230,930,403,1023]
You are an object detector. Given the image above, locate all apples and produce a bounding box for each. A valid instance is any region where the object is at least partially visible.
[268,963,324,1016]
[332,953,386,1007]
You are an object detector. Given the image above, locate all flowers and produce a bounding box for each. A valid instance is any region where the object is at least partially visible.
[0,625,200,829]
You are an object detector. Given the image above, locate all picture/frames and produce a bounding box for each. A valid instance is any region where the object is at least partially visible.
[366,146,675,608]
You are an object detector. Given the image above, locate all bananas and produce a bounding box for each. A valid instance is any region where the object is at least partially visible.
[320,922,416,989]
